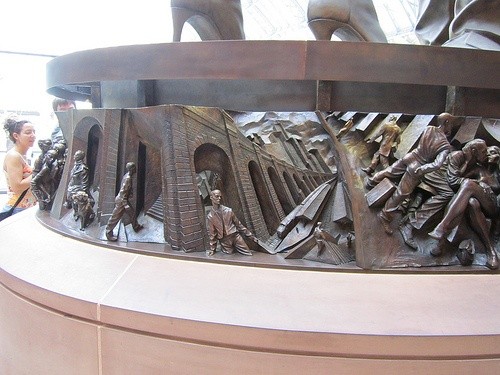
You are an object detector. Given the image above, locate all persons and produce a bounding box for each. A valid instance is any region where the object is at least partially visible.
[67,151,95,221]
[365,112,500,270]
[30,136,68,211]
[105,162,144,243]
[337,116,355,141]
[51,98,77,140]
[206,189,258,256]
[4,120,38,214]
[363,116,401,177]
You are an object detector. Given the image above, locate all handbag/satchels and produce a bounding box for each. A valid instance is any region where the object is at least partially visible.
[0,209,13,222]
